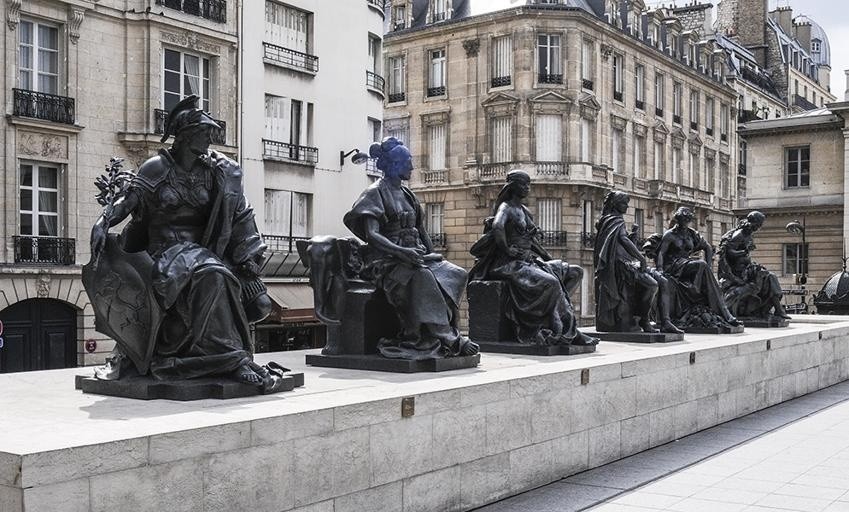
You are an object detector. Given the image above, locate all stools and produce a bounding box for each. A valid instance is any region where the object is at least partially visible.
[593,278,641,331]
[468,279,513,342]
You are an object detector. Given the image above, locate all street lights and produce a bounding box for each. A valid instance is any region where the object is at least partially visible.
[786,219,806,315]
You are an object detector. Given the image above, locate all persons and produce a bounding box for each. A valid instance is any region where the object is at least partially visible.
[89,94,273,385]
[718,210,792,321]
[470,169,601,347]
[728,229,751,276]
[343,135,480,360]
[591,190,686,333]
[656,207,744,325]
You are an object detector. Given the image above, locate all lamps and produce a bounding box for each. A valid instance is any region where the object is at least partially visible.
[340,149,368,166]
[785,216,806,242]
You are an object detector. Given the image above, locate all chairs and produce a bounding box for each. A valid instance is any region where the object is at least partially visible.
[294,233,399,356]
